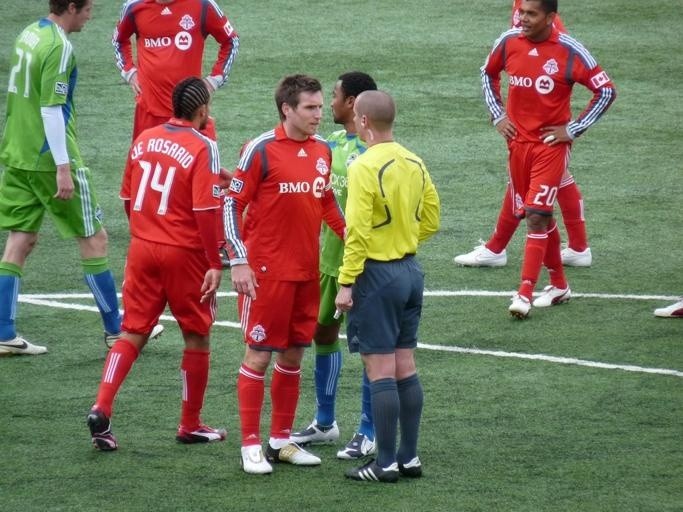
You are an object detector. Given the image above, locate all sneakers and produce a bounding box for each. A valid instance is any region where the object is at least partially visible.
[87,405,117,450]
[0,337,47,355]
[454,238,592,319]
[104,324,164,348]
[177,421,421,483]
[654,303,683,318]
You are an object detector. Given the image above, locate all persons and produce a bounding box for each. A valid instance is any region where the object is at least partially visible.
[481,0,617,319]
[112,0,240,147]
[334,90,439,484]
[654,297,683,318]
[288,71,378,458]
[0,1,165,358]
[223,75,346,475]
[88,78,227,453]
[454,0,593,266]
